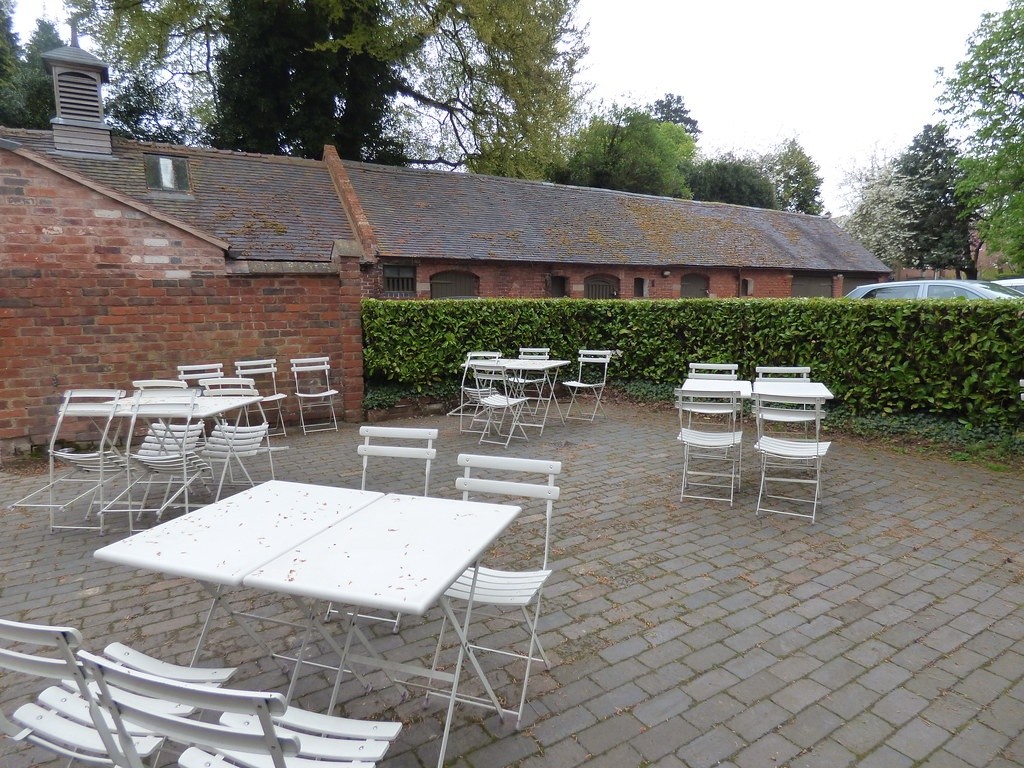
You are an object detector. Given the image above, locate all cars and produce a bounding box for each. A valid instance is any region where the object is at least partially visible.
[990,279,1024,296]
[844,280,1024,301]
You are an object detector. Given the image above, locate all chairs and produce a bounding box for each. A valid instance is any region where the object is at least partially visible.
[0,348,834,768]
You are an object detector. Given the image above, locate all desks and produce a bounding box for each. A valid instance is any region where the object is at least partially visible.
[117,390,274,523]
[93,478,384,765]
[507,360,571,437]
[243,493,521,768]
[48,388,194,521]
[461,358,515,437]
[753,381,834,506]
[681,379,753,493]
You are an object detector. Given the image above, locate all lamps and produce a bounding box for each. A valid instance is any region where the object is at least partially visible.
[700,288,709,293]
[595,279,617,296]
